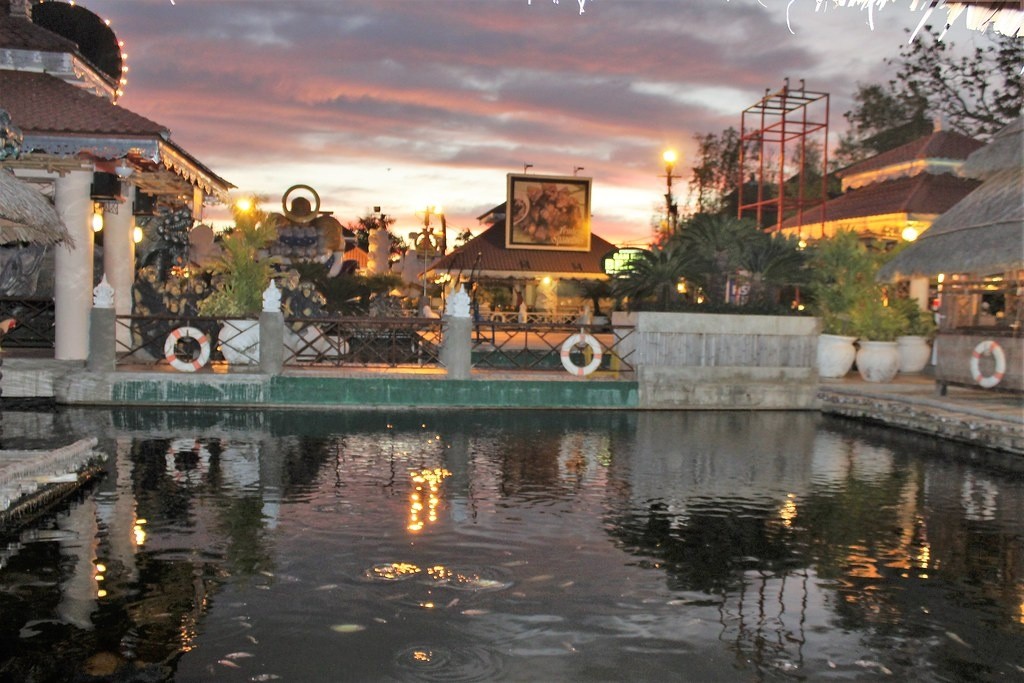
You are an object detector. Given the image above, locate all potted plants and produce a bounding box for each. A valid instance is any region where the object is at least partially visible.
[601,214,820,380]
[808,225,935,383]
[198,196,278,365]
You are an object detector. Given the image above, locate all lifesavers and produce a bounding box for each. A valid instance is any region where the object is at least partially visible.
[560,332,604,376]
[164,326,212,373]
[969,339,1006,389]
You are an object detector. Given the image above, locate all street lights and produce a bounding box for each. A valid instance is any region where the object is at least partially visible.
[664,149,678,235]
